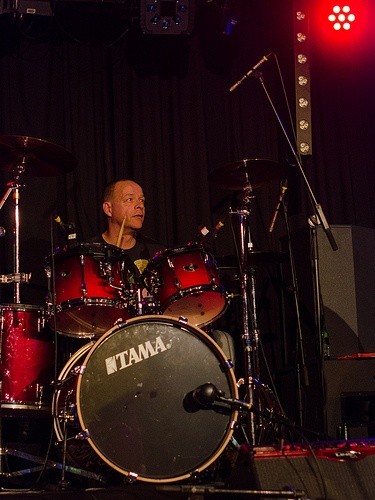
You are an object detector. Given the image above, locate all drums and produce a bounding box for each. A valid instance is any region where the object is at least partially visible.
[48,241,126,337]
[73,316,241,483]
[148,243,226,330]
[0,305,57,411]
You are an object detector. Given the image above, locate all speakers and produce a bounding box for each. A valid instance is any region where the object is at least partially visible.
[314,223,375,442]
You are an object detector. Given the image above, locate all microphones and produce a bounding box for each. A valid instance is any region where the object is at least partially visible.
[229,51,272,92]
[53,212,79,253]
[201,216,227,243]
[194,381,256,412]
[269,179,288,233]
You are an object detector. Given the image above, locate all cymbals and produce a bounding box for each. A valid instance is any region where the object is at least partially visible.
[213,160,281,190]
[4,135,70,178]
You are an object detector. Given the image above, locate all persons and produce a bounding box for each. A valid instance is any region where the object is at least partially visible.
[54,177,158,299]
